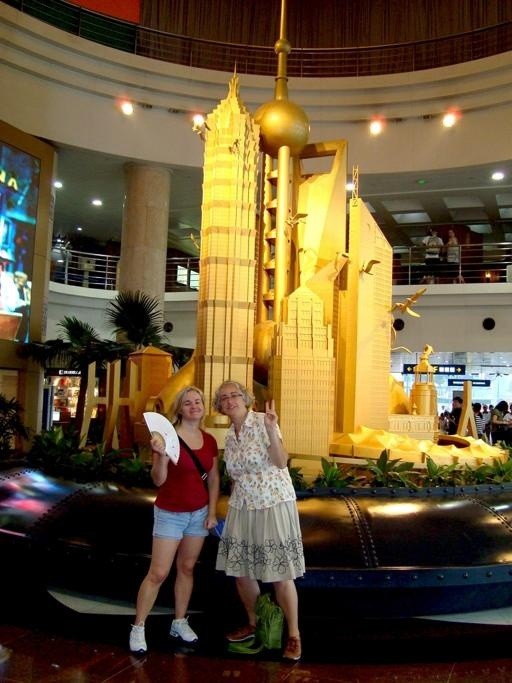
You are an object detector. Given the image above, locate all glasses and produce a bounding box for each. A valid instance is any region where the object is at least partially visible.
[220,393,243,401]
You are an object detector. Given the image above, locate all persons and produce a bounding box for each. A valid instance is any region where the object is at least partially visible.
[439,413,445,431]
[444,412,449,426]
[482,405,491,441]
[472,403,485,439]
[421,228,445,284]
[446,397,463,435]
[212,381,305,660]
[491,401,512,445]
[129,385,219,652]
[503,403,512,447]
[489,405,494,411]
[446,229,465,283]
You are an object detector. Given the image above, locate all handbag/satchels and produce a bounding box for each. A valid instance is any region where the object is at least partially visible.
[178,434,224,538]
[228,594,284,655]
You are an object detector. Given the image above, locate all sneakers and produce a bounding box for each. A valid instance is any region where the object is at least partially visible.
[170,616,198,644]
[282,636,302,660]
[130,622,147,652]
[227,624,255,641]
